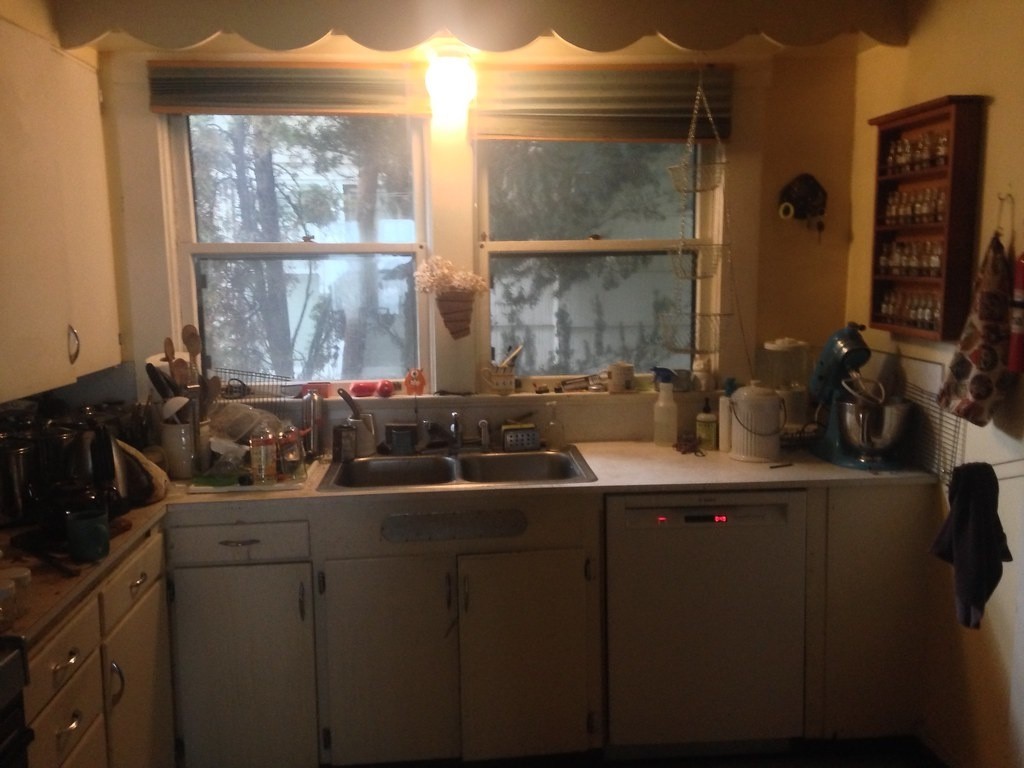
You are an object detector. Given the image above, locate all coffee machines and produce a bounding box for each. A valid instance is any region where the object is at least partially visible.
[764,337,808,436]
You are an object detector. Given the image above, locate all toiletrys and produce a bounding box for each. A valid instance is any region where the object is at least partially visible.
[544,400,566,453]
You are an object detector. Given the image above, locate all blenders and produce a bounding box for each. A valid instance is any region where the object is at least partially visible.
[810,327,914,470]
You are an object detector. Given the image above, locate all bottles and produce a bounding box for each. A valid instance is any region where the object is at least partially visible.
[302,388,322,457]
[249,430,308,487]
[719,378,736,452]
[696,398,716,449]
[0,568,33,633]
[881,129,950,332]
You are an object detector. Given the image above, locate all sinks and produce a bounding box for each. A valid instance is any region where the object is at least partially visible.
[316,454,463,492]
[453,443,598,486]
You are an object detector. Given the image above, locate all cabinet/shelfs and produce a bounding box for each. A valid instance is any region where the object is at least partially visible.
[809,487,931,742]
[1,19,121,405]
[25,486,607,768]
[868,90,985,344]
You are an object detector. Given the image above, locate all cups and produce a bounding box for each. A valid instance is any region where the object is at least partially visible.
[481,365,515,395]
[598,362,634,394]
[333,424,357,463]
[159,416,211,479]
[213,453,244,482]
[392,428,412,456]
[348,413,378,458]
[67,511,110,563]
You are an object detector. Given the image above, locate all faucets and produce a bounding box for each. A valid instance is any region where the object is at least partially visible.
[421,419,459,457]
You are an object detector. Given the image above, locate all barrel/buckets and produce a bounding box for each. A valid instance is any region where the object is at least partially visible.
[1,396,140,526]
[728,379,788,464]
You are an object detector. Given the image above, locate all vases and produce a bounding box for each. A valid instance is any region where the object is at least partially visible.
[436,292,473,340]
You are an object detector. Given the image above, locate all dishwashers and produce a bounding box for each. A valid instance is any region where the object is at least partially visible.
[605,488,808,745]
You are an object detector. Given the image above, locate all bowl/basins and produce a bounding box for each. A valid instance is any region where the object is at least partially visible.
[212,402,284,445]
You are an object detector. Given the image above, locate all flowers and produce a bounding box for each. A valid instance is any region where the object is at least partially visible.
[414,255,493,295]
